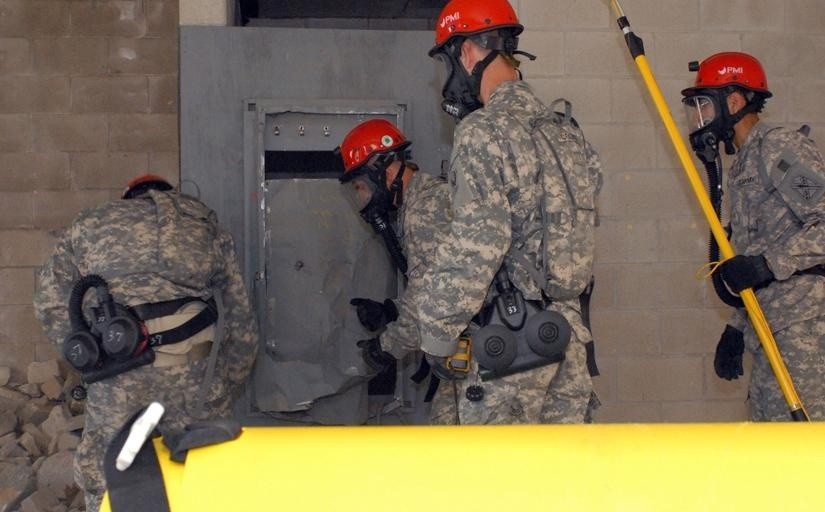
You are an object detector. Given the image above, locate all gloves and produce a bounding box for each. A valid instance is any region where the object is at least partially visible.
[720,254,775,294]
[714,324,745,380]
[350,299,399,332]
[357,335,395,372]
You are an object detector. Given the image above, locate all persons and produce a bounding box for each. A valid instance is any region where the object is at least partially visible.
[336,117,456,377]
[30,172,262,512]
[679,49,822,422]
[412,0,602,422]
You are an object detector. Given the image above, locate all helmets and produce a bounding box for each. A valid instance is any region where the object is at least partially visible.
[429,0,524,57]
[681,52,772,98]
[339,119,412,184]
[121,174,173,199]
